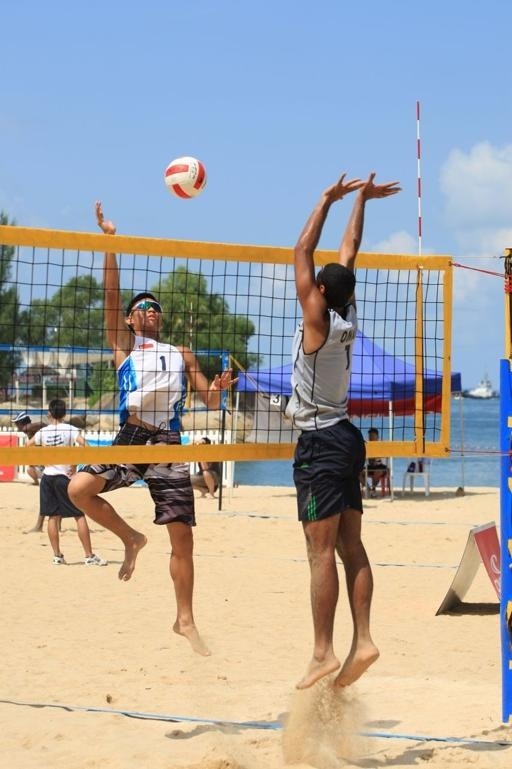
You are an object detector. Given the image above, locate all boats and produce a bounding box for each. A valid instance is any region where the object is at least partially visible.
[463,379,499,399]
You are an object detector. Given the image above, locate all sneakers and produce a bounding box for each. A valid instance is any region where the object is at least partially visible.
[84,553,109,567]
[52,553,67,565]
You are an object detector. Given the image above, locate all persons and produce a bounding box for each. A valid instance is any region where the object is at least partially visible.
[11,411,94,534]
[189,437,220,500]
[290,172,404,694]
[22,399,108,566]
[359,427,388,499]
[65,198,240,659]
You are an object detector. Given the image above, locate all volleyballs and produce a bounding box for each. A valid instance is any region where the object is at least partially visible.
[165,157,207,199]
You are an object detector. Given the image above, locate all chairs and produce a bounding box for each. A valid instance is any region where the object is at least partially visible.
[361,451,430,501]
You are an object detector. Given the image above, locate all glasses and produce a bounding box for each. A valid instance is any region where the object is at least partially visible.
[133,301,164,314]
